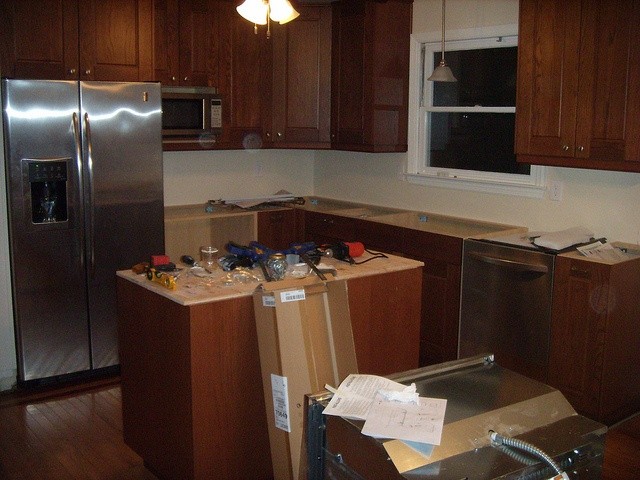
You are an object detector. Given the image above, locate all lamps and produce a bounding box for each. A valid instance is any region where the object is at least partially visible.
[428,1,457,81]
[235,1,301,40]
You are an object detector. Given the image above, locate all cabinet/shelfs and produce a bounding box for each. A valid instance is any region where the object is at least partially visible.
[513,0,640,156]
[549,255,640,427]
[219,0,332,143]
[332,0,410,147]
[355,218,462,367]
[155,0,219,90]
[296,209,355,245]
[0,0,155,82]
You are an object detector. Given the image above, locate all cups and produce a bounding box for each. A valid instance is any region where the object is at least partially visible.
[201,246,218,270]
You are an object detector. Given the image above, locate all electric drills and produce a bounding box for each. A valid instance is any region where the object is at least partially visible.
[308,241,365,265]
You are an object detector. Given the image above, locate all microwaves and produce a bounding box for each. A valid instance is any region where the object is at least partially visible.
[162,86,223,145]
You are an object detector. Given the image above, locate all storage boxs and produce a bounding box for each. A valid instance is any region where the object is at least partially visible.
[252,273,362,479]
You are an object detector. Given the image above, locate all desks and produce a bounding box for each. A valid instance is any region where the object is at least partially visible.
[114,244,424,478]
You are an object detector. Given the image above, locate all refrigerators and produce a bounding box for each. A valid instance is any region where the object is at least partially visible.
[1,76,165,382]
